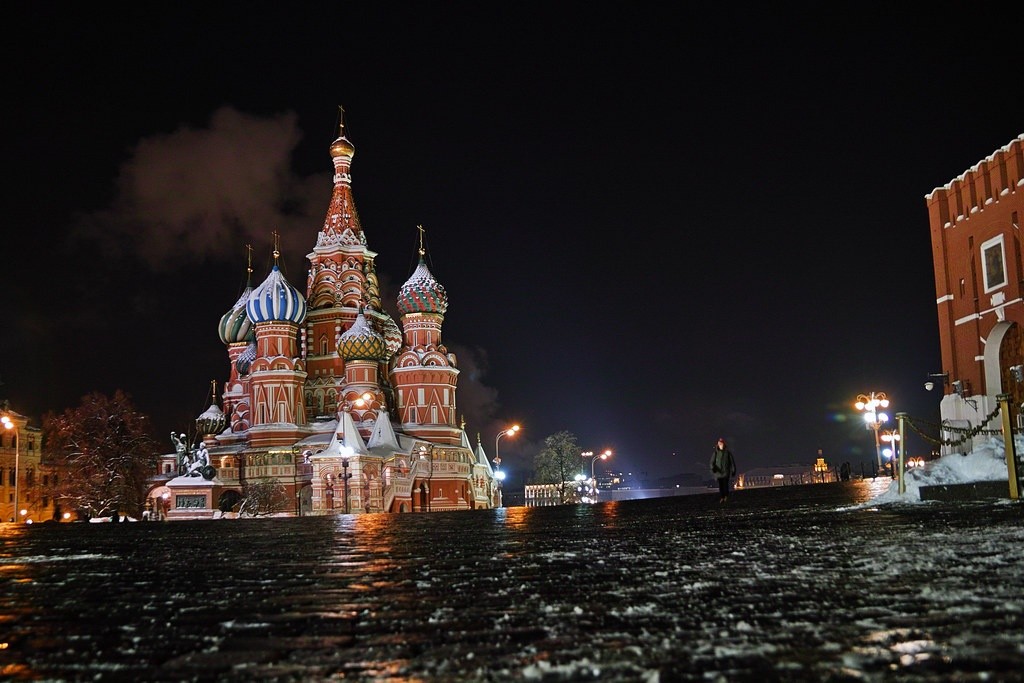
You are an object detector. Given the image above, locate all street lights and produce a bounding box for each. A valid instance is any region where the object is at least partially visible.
[494,470,507,508]
[905,456,925,469]
[493,424,520,471]
[880,429,900,479]
[591,450,612,502]
[854,391,889,476]
[1,416,19,522]
[340,447,354,514]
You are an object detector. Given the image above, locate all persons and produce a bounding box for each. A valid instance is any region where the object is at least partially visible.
[156,496,167,521]
[170,431,191,476]
[184,443,209,477]
[142,497,154,520]
[709,439,735,502]
